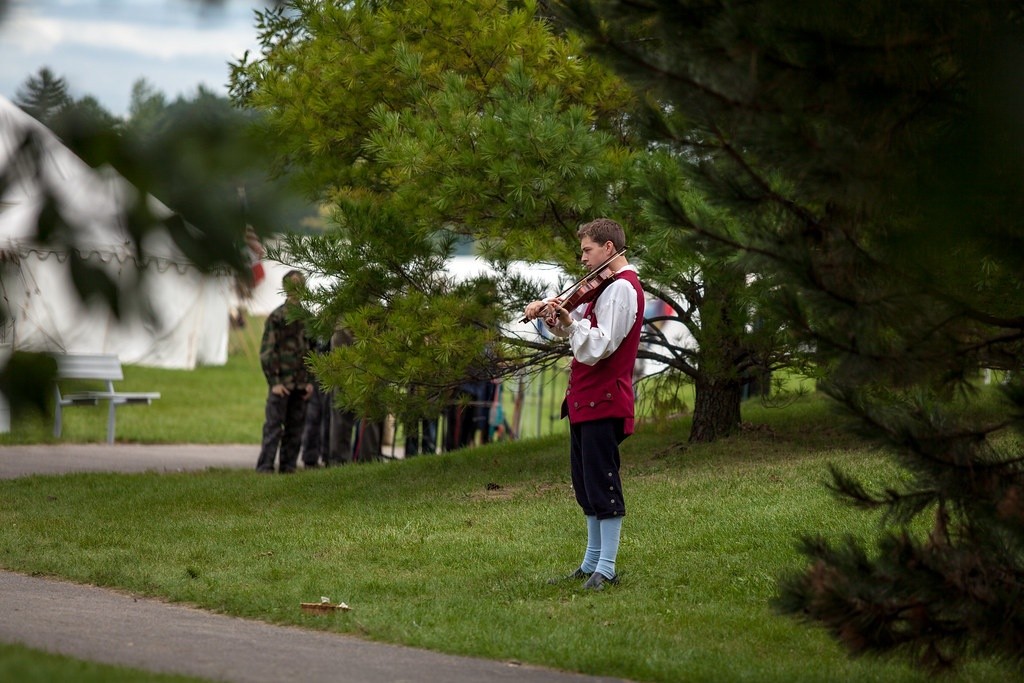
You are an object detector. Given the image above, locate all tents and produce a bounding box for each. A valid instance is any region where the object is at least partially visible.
[0,97,231,370]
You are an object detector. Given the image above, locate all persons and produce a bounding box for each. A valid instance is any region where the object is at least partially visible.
[236,226,266,324]
[259,271,495,476]
[526,218,644,587]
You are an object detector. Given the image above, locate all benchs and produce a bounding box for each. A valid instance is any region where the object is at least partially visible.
[50,354,161,444]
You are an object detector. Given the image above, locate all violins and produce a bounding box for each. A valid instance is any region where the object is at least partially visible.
[545,267,615,328]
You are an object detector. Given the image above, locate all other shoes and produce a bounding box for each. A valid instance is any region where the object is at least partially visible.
[581,572,620,593]
[547,568,594,584]
